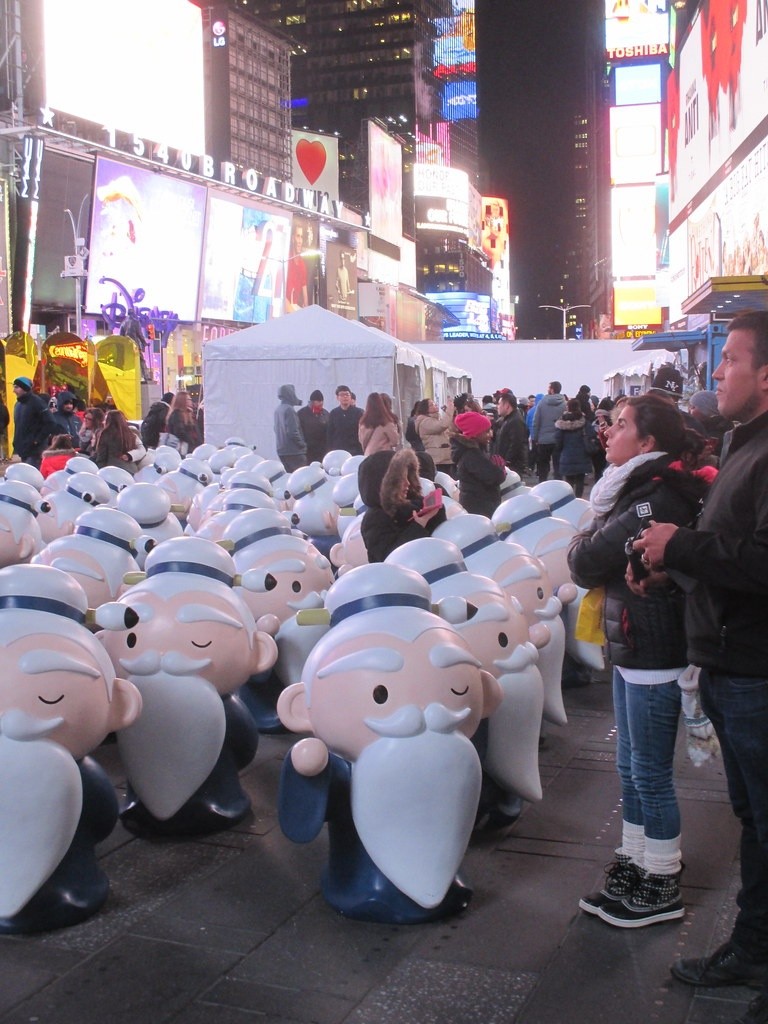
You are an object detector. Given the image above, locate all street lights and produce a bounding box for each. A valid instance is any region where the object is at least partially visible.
[537,305,592,340]
[62,192,98,341]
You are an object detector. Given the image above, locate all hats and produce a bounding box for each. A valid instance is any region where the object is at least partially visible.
[454,412,491,439]
[310,390,324,401]
[454,392,468,409]
[649,366,684,399]
[277,384,303,405]
[691,390,719,414]
[14,376,32,392]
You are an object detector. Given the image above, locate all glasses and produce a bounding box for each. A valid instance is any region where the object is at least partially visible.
[83,417,92,422]
[63,400,74,405]
[338,393,353,397]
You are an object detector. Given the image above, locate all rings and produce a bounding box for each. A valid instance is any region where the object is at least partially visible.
[641,554,648,563]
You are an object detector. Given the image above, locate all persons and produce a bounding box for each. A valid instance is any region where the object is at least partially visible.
[449,377,614,498]
[285,219,310,307]
[233,208,277,324]
[272,383,311,473]
[13,376,146,477]
[323,385,452,474]
[624,310,768,991]
[296,387,331,463]
[335,250,356,316]
[567,392,736,929]
[0,444,606,925]
[356,450,449,567]
[448,419,507,520]
[141,392,204,459]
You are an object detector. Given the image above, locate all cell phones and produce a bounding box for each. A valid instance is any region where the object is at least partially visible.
[409,487,443,521]
[447,396,450,399]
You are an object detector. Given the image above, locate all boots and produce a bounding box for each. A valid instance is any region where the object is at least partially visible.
[578,847,640,916]
[597,861,686,929]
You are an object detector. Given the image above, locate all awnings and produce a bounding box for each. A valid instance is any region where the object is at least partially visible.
[631,326,709,389]
[679,277,768,316]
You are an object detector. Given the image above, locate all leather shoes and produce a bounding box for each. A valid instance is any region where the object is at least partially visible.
[734,995,768,1024]
[671,940,768,990]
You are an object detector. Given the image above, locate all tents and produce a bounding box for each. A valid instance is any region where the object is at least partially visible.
[602,350,676,399]
[199,304,473,461]
